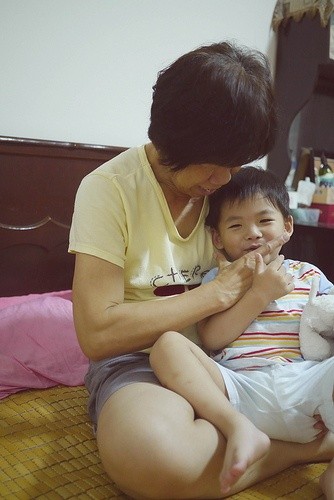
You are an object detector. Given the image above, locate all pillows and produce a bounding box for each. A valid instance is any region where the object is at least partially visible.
[0,290,89,399]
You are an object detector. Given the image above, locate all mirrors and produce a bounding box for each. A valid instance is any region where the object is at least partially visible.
[266,0,334,192]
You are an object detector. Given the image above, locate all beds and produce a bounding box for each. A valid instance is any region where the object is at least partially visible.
[0,136,330,500]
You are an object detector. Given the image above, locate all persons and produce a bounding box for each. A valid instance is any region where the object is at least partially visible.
[68,41,333,500]
[147,166,333,497]
